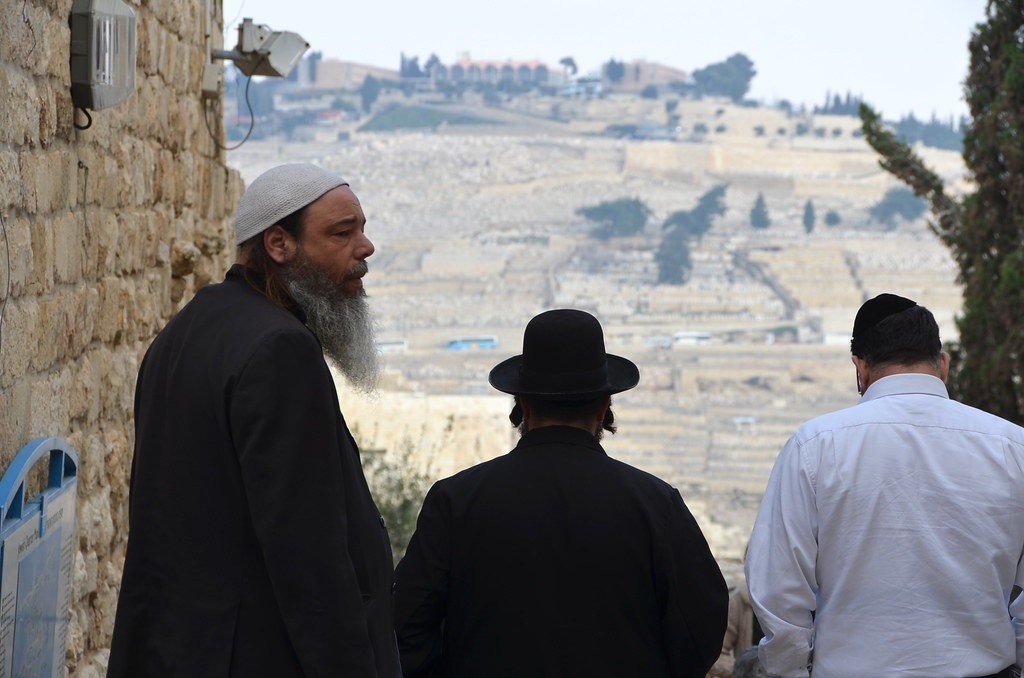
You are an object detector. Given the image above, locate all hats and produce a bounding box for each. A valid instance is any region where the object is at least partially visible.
[488,309,641,402]
[231,164,349,247]
[851,294,916,338]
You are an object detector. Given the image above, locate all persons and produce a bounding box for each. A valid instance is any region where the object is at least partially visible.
[108,163,401,677]
[740,295,1024,678]
[722,547,765,664]
[394,309,730,678]
